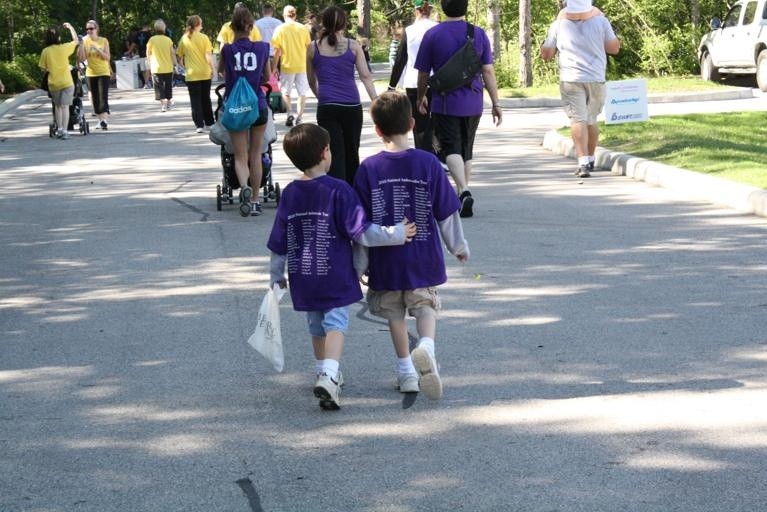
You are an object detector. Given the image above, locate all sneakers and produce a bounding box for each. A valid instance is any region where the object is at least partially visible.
[144,83,151,89]
[57,119,108,140]
[162,102,175,111]
[239,186,256,218]
[286,113,303,126]
[250,202,262,216]
[459,191,474,217]
[313,343,442,409]
[196,125,213,133]
[575,161,594,178]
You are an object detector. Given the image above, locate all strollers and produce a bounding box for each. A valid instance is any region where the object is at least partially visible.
[212,83,282,209]
[46,68,90,137]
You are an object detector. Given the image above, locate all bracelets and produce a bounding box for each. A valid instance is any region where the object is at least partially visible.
[493,105,500,108]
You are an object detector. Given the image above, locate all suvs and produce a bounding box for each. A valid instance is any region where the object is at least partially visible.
[696,1,767,93]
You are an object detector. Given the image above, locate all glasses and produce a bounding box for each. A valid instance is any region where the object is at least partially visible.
[85,27,95,31]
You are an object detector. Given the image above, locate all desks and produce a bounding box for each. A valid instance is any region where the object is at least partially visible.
[115,58,144,90]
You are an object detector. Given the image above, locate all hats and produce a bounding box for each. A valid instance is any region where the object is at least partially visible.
[441,0,468,16]
[415,0,434,9]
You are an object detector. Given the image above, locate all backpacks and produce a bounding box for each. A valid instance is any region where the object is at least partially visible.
[221,42,263,132]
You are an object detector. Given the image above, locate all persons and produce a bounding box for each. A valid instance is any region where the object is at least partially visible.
[37,20,80,141]
[352,88,472,404]
[557,0,605,21]
[361,36,371,73]
[78,20,115,132]
[68,33,92,100]
[413,0,505,221]
[265,120,419,412]
[388,30,401,67]
[109,2,315,139]
[307,5,379,181]
[540,15,624,178]
[218,6,272,217]
[387,0,449,173]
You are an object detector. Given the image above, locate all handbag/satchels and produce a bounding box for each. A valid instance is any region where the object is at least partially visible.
[428,41,482,96]
